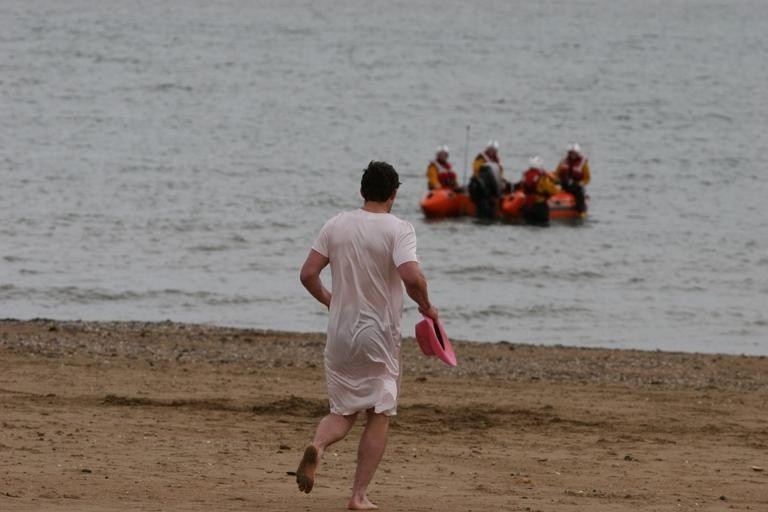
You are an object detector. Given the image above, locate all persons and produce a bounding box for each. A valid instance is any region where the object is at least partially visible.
[426,144,458,190]
[473,140,512,190]
[517,153,553,195]
[295,159,440,511]
[555,145,591,216]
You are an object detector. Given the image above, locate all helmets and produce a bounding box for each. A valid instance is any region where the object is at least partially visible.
[531,157,544,173]
[487,141,499,152]
[568,142,581,156]
[436,144,449,155]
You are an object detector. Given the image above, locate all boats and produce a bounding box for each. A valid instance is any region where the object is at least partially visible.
[418,187,590,224]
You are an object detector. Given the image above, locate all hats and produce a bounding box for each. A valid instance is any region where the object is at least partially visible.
[415,307,457,367]
